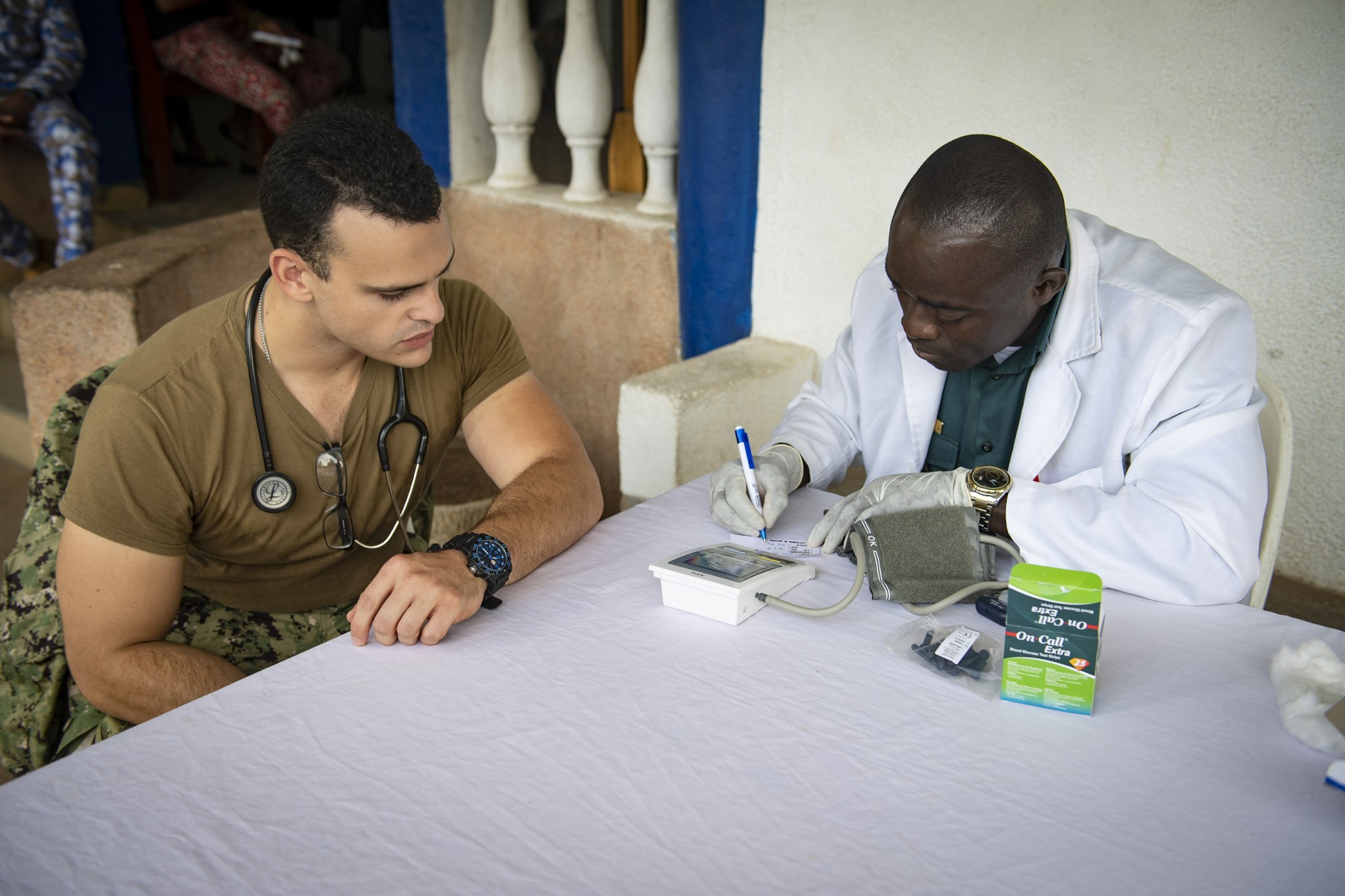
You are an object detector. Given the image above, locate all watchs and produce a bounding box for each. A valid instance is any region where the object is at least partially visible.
[964,465,1012,536]
[436,530,514,611]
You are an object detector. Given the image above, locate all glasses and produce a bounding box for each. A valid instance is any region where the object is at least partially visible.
[315,442,355,551]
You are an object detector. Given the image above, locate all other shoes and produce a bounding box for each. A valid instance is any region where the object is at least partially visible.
[22,237,58,280]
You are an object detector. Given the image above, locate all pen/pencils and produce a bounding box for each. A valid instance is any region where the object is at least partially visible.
[735,426,766,543]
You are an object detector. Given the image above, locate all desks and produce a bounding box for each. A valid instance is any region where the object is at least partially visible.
[2,467,1341,892]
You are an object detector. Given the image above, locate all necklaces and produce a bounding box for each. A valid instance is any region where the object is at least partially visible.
[259,281,277,373]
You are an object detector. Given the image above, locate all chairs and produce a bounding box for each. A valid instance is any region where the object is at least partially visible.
[1250,376,1294,609]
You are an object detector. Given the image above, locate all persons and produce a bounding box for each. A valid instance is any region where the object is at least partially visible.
[1,1,347,275]
[705,133,1270,607]
[54,100,606,726]
